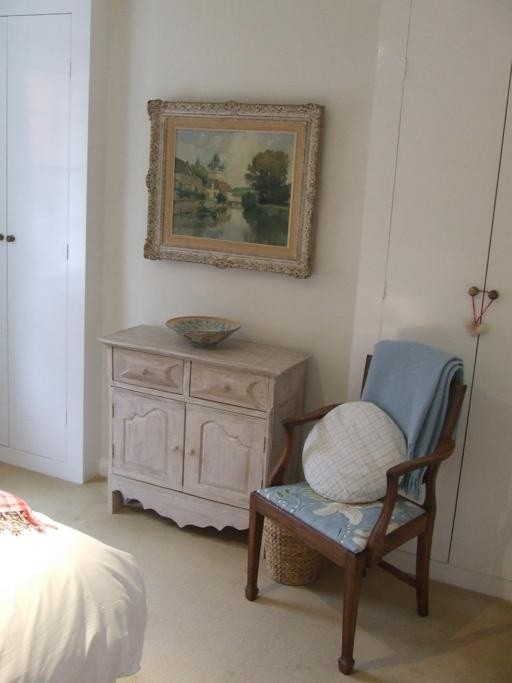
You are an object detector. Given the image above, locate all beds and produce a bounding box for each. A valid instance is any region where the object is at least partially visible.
[0,484,145,683]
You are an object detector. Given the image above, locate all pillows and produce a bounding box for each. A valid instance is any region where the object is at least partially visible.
[300,400,408,504]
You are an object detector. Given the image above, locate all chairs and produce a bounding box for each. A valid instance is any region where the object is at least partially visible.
[244,353,468,675]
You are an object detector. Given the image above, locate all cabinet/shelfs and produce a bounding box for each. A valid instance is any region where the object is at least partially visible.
[98,323,312,559]
[1,0,105,482]
[346,1,511,600]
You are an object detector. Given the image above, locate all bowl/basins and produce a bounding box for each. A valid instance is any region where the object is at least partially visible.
[165,315,243,350]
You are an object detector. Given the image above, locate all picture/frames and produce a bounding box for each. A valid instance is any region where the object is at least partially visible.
[141,96,326,276]
